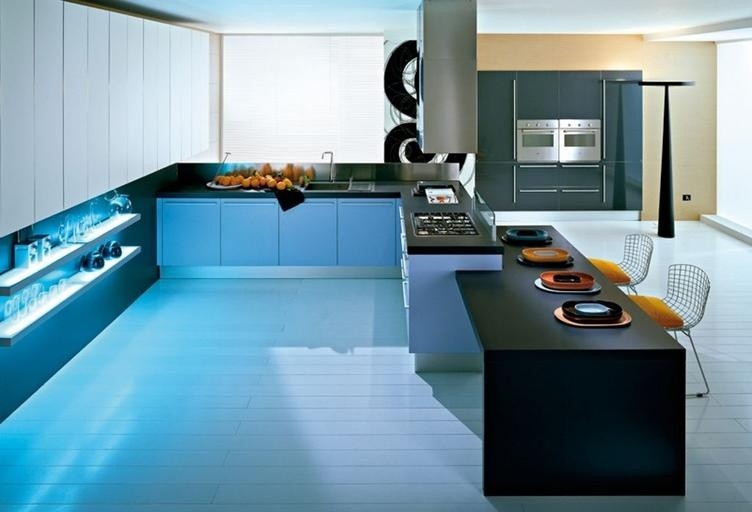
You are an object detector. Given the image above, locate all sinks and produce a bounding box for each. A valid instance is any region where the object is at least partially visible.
[300,181,352,193]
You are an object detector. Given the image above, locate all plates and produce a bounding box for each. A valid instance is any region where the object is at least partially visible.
[563,299,622,323]
[206,181,241,189]
[523,246,569,263]
[501,234,557,246]
[516,252,576,268]
[540,270,596,291]
[505,228,550,243]
[531,277,603,294]
[553,305,633,328]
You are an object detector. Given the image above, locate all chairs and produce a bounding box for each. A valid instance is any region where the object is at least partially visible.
[587,233,655,296]
[624,264,712,397]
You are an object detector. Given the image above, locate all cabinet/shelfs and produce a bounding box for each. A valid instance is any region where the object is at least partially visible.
[190,29,221,167]
[107,10,145,194]
[0,0,65,240]
[169,26,193,169]
[394,199,507,374]
[62,2,112,211]
[277,199,398,269]
[151,195,280,269]
[1,211,143,349]
[142,17,171,181]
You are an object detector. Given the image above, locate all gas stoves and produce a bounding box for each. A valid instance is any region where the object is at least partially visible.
[410,211,481,240]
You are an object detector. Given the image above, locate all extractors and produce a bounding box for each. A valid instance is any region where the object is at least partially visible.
[414,0,479,155]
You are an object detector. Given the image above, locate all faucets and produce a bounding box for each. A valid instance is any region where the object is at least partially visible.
[321,151,336,182]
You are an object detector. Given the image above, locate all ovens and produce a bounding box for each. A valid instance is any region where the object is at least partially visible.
[516,119,557,164]
[559,118,602,165]
